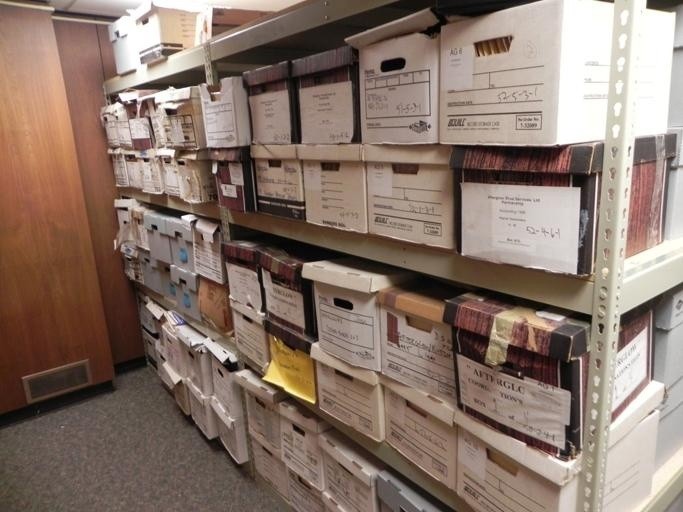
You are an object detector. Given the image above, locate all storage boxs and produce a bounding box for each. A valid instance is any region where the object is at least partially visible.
[102,1,682,512]
